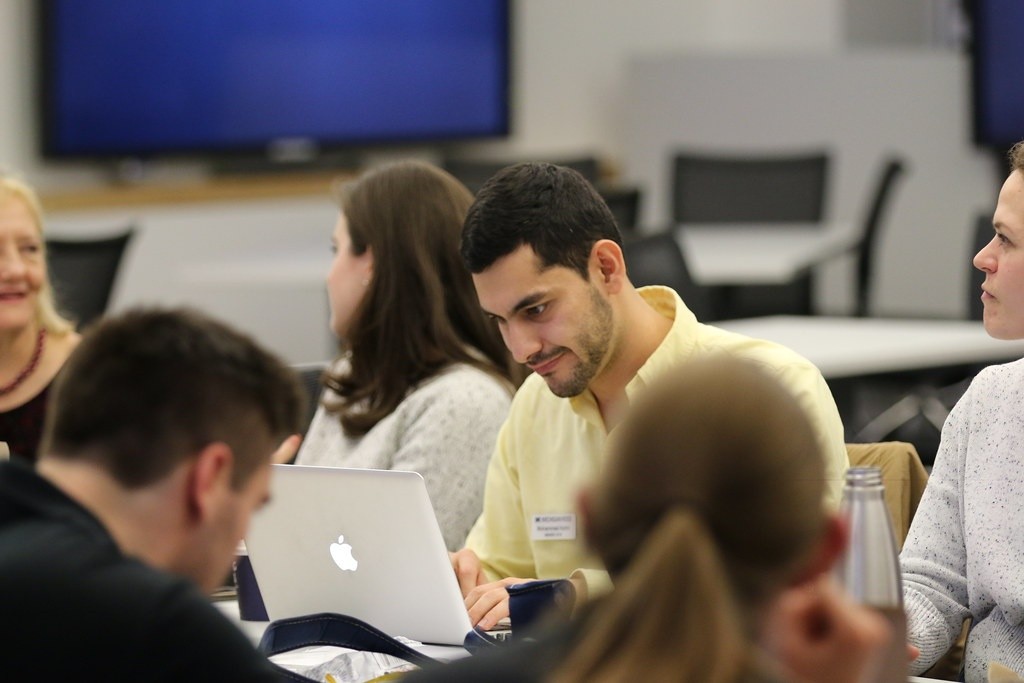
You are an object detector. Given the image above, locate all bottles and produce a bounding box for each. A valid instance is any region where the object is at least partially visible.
[829,466,905,610]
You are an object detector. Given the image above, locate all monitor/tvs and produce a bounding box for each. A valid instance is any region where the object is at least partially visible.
[33,0,516,186]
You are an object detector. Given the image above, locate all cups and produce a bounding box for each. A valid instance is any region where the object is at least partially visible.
[506,578,578,642]
[232,542,269,622]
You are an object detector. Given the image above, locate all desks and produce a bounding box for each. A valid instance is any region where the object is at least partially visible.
[708,316,1024,446]
[664,214,867,321]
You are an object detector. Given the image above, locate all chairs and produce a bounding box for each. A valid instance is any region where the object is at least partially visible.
[621,151,906,326]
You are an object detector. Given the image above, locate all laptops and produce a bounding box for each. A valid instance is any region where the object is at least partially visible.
[244,464,516,645]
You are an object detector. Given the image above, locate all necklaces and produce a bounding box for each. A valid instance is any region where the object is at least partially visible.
[0,328,48,397]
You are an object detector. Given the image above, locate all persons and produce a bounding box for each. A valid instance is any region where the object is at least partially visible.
[0,178,83,470]
[364,350,894,683]
[870,137,1024,683]
[0,306,311,683]
[447,162,854,633]
[289,159,523,557]
